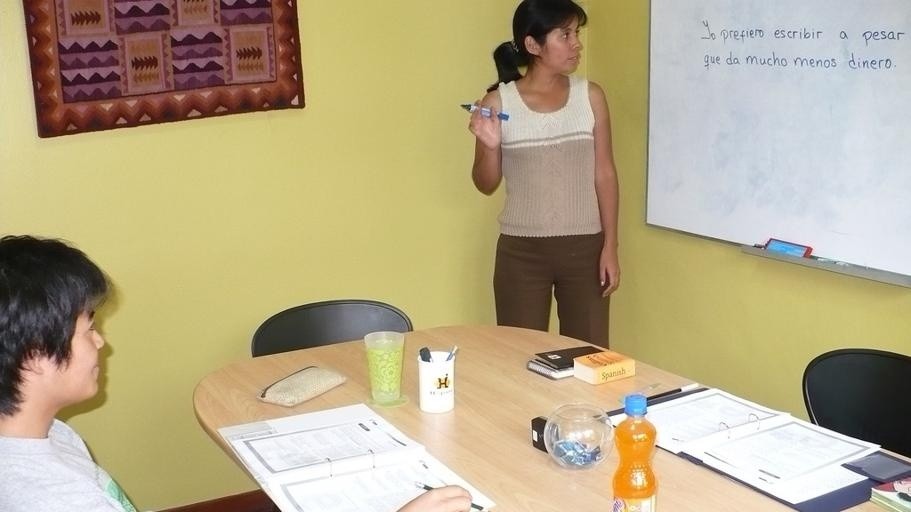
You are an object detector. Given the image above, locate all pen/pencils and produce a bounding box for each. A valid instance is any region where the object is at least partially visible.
[416,483,483,511]
[446,346,458,360]
[647,383,699,400]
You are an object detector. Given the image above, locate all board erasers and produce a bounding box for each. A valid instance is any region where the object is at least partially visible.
[764,238,812,258]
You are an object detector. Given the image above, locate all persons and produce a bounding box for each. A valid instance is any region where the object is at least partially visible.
[468,2,622,349]
[0,233,475,512]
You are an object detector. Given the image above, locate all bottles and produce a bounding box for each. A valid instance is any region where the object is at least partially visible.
[610,396,658,512]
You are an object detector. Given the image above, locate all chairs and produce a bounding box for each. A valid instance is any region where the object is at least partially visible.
[801,348,911,460]
[251,298,412,356]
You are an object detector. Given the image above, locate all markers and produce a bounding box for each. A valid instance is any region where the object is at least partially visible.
[460,104,509,121]
[420,347,432,362]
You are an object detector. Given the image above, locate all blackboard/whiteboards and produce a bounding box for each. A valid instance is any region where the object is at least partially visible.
[646,0,911,288]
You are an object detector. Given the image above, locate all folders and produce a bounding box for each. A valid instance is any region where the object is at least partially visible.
[606,389,911,512]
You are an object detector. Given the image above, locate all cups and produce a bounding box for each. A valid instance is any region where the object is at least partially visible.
[417,349,458,414]
[363,329,406,405]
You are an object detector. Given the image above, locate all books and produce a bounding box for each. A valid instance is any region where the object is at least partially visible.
[870,474,911,512]
[868,496,903,512]
[527,358,575,381]
[533,345,607,370]
[573,348,636,387]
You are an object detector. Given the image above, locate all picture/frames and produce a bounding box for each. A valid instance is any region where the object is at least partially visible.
[22,0,305,138]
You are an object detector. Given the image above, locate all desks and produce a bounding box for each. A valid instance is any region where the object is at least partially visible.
[192,325,911,512]
